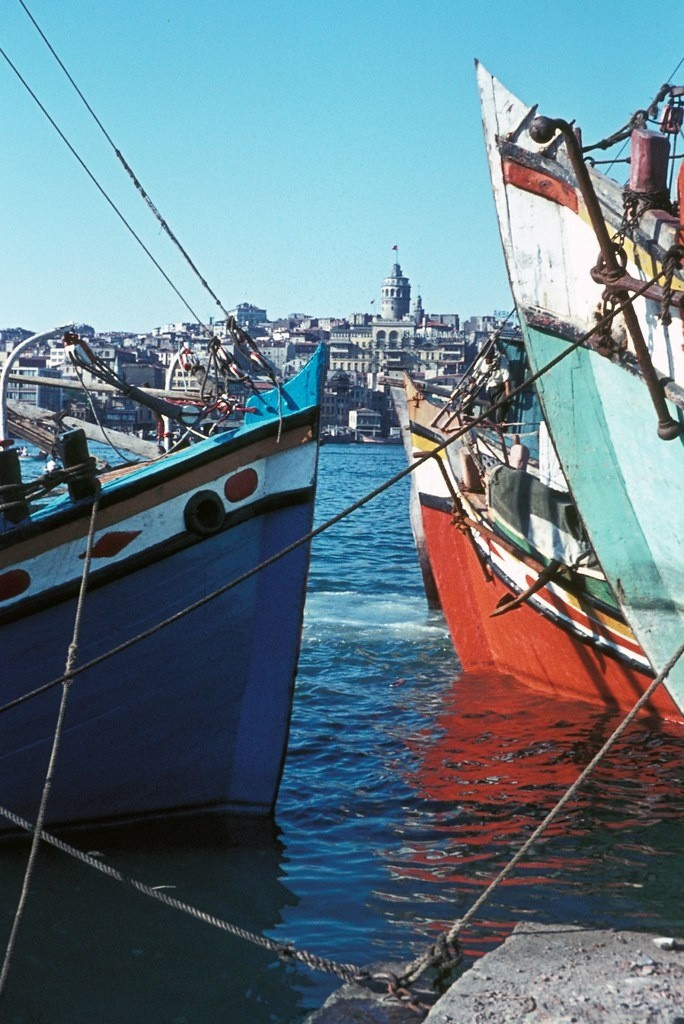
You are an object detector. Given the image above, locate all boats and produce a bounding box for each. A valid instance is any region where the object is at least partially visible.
[381,322,684,730]
[472,52,684,726]
[1,0,329,841]
